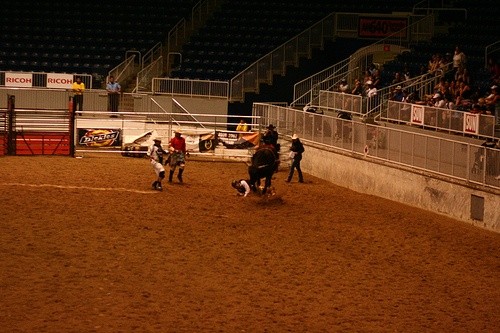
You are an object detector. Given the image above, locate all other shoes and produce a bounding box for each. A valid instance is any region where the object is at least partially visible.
[151,181,163,191]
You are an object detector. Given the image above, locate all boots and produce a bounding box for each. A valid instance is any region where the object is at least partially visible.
[169,169,174,182]
[177,168,184,183]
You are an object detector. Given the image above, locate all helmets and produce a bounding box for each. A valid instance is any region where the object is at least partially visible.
[232,180,237,187]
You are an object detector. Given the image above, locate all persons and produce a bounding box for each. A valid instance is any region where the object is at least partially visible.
[72,77,85,116]
[232,120,305,197]
[165,130,186,184]
[149,138,164,192]
[340,46,500,182]
[106,76,121,118]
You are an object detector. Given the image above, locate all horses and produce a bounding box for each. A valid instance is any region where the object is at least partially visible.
[248,145,278,199]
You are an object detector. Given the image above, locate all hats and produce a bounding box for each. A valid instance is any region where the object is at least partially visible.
[366,80,372,85]
[292,133,299,139]
[266,124,276,130]
[173,129,182,134]
[491,85,499,90]
[432,94,440,98]
[152,137,161,142]
